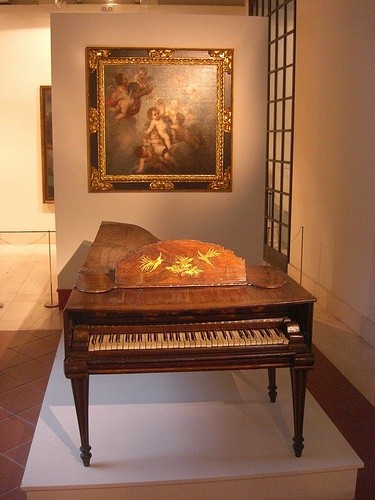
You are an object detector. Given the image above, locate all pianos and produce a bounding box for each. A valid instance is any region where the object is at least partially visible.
[61,219,320,468]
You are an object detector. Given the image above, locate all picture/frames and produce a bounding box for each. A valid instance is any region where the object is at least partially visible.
[39,86,54,203]
[85,47,234,193]
